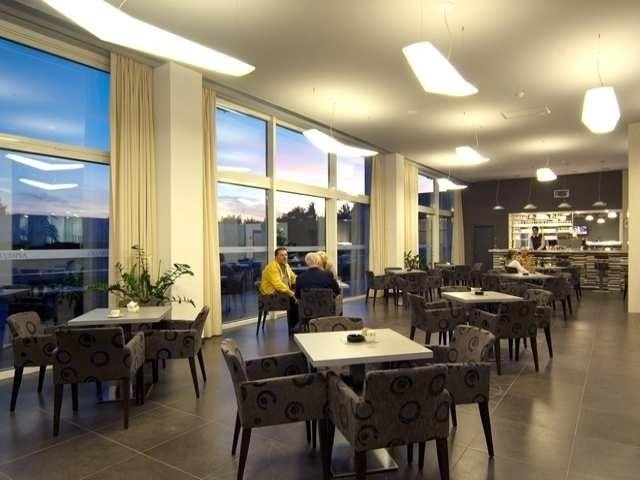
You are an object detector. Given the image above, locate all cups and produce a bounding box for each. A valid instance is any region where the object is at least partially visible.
[471,287,480,294]
[111,310,121,314]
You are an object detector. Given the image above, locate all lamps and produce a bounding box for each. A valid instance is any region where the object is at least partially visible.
[591,160,607,207]
[579,32,620,132]
[47,0,259,78]
[533,159,557,183]
[523,176,538,210]
[555,162,573,209]
[454,129,490,169]
[302,101,381,159]
[491,173,506,211]
[401,12,480,98]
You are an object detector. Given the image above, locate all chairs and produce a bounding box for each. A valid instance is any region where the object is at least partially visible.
[7,311,66,411]
[52,327,145,437]
[255,281,370,374]
[410,324,495,457]
[363,256,584,376]
[221,254,351,313]
[144,304,210,398]
[7,266,110,324]
[220,338,329,479]
[116,299,166,370]
[326,363,451,479]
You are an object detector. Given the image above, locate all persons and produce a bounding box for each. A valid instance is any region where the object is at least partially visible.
[295,251,342,318]
[529,226,547,250]
[502,249,531,273]
[579,238,591,249]
[316,251,337,281]
[259,245,298,339]
[515,245,535,274]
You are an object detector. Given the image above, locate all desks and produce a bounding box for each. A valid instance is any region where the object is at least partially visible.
[293,327,433,478]
[67,306,173,404]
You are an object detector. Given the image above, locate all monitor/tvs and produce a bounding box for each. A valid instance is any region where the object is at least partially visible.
[574,224,589,235]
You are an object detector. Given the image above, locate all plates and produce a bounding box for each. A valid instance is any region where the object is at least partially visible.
[109,315,125,317]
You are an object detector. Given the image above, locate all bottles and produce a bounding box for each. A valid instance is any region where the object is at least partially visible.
[363,328,378,343]
